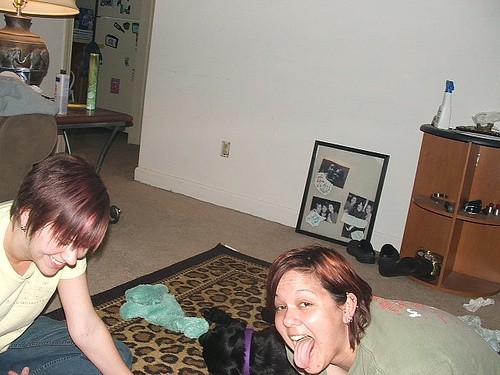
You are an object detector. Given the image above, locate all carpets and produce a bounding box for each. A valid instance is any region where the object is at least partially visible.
[42,244,273,375]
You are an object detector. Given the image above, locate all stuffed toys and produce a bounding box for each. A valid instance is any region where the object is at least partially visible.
[119,284,209,339]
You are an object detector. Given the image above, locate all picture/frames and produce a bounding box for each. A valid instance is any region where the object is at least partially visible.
[295,140,390,246]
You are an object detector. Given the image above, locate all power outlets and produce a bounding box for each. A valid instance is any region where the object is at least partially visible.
[220,141,230,158]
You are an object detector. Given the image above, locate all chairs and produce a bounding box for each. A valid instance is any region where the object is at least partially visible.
[0,71,59,205]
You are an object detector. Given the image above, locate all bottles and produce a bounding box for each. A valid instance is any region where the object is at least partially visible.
[54,69,70,115]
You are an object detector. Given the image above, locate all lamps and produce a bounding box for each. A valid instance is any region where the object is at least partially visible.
[0,0,82,88]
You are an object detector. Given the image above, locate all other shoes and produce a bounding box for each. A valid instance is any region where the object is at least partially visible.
[347,238,376,264]
[378,243,400,264]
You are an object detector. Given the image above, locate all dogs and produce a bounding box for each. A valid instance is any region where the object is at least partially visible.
[198,307,302,375]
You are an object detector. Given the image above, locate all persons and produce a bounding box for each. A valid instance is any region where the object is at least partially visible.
[313,163,371,225]
[0,152,139,375]
[266,243,500,375]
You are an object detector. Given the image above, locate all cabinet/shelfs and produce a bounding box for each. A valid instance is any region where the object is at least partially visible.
[399,124,500,297]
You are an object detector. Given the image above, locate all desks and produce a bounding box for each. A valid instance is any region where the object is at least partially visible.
[55,103,134,224]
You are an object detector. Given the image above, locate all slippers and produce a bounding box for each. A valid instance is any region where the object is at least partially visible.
[379,256,434,278]
